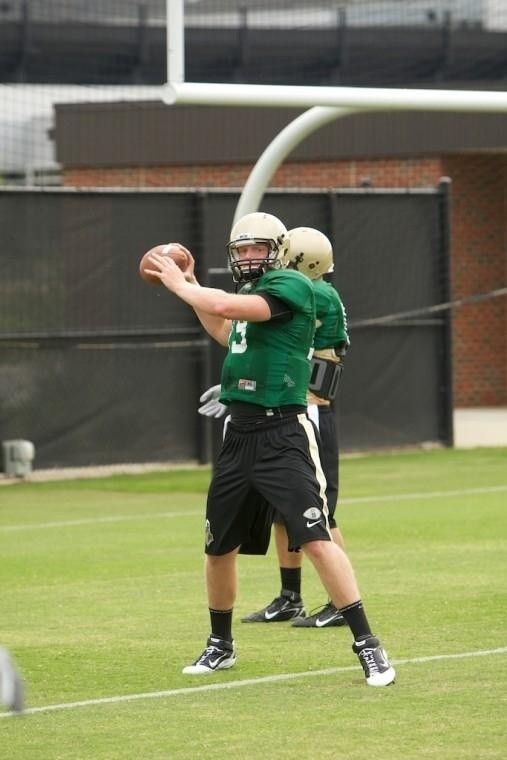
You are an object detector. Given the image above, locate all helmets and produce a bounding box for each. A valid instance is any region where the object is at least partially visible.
[225,211,291,282]
[277,226,335,281]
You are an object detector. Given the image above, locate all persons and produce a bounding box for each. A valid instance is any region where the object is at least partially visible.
[139,210,397,686]
[193,222,350,628]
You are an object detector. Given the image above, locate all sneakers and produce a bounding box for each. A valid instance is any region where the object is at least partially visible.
[239,595,307,622]
[181,631,237,674]
[352,634,396,687]
[291,600,347,627]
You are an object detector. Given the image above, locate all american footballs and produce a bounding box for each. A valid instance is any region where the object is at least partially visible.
[138,243,189,286]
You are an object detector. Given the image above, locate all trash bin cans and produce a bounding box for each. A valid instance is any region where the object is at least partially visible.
[2,440,35,478]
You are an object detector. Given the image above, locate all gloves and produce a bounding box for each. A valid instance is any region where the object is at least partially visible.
[197,383,228,419]
